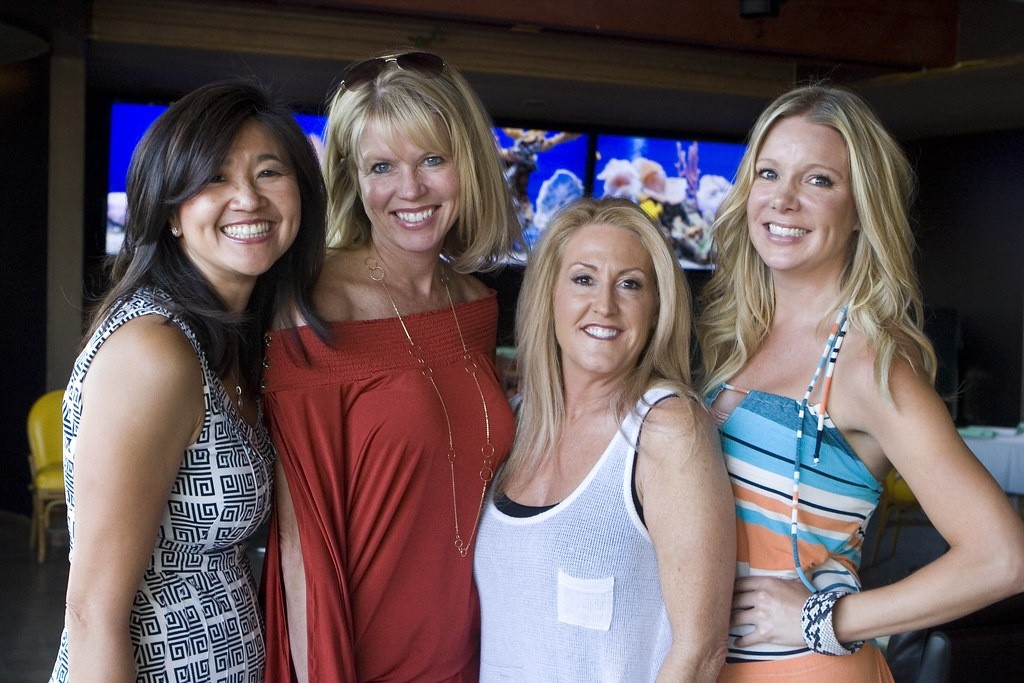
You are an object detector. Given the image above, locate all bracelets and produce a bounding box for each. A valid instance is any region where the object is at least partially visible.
[802,589,865,656]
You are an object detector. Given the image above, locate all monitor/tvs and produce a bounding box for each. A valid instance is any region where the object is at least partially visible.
[102,100,347,259]
[466,119,755,276]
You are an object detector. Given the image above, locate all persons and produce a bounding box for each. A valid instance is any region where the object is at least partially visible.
[683,81,1024,683]
[469,195,740,683]
[39,72,332,683]
[258,45,518,683]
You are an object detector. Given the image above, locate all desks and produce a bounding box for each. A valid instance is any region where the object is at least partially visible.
[961,425,1024,495]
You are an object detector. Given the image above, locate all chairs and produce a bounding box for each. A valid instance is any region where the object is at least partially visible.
[865,473,920,569]
[30,390,67,567]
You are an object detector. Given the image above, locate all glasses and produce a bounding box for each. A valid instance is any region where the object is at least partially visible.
[340,52,449,92]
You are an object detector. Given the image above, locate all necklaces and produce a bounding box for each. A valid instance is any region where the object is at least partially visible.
[364,241,497,558]
[230,369,244,410]
